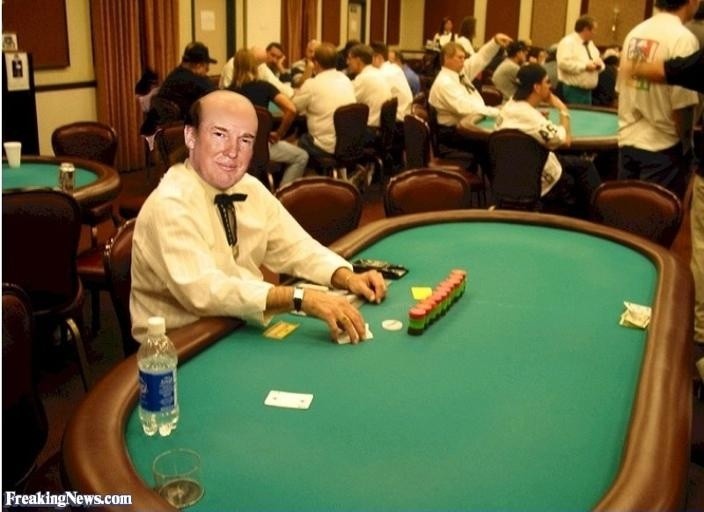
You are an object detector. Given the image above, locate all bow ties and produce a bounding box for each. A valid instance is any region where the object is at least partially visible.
[214,193,248,246]
[583,42,594,61]
[459,74,476,94]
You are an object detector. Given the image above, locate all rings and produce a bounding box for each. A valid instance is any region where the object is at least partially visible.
[340,316,348,324]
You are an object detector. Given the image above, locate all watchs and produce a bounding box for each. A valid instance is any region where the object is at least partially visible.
[293,285,304,312]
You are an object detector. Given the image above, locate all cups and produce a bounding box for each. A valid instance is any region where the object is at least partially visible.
[153,448,202,505]
[4,142,22,168]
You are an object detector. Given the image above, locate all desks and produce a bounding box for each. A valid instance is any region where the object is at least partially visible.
[464,103,620,147]
[71,208,692,512]
[2,154,117,211]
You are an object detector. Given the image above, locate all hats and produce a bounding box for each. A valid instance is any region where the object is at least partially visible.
[183,42,218,64]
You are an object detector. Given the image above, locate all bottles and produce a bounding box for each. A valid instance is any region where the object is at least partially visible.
[60,162,74,196]
[137,315,179,436]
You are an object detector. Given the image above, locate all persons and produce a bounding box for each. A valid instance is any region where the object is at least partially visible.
[219,1,702,215]
[127,89,386,352]
[155,39,218,125]
[620,43,704,386]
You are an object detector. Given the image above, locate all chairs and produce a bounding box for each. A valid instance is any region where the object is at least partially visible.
[383,169,468,216]
[270,176,361,247]
[3,283,51,486]
[582,178,681,249]
[413,105,483,175]
[150,94,182,125]
[78,235,114,338]
[252,105,275,189]
[120,127,187,219]
[478,128,544,215]
[403,114,482,202]
[476,87,504,109]
[302,104,367,187]
[51,122,118,250]
[369,96,398,183]
[2,186,96,391]
[102,217,142,357]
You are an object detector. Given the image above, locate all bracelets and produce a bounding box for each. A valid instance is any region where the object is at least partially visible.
[344,272,353,290]
